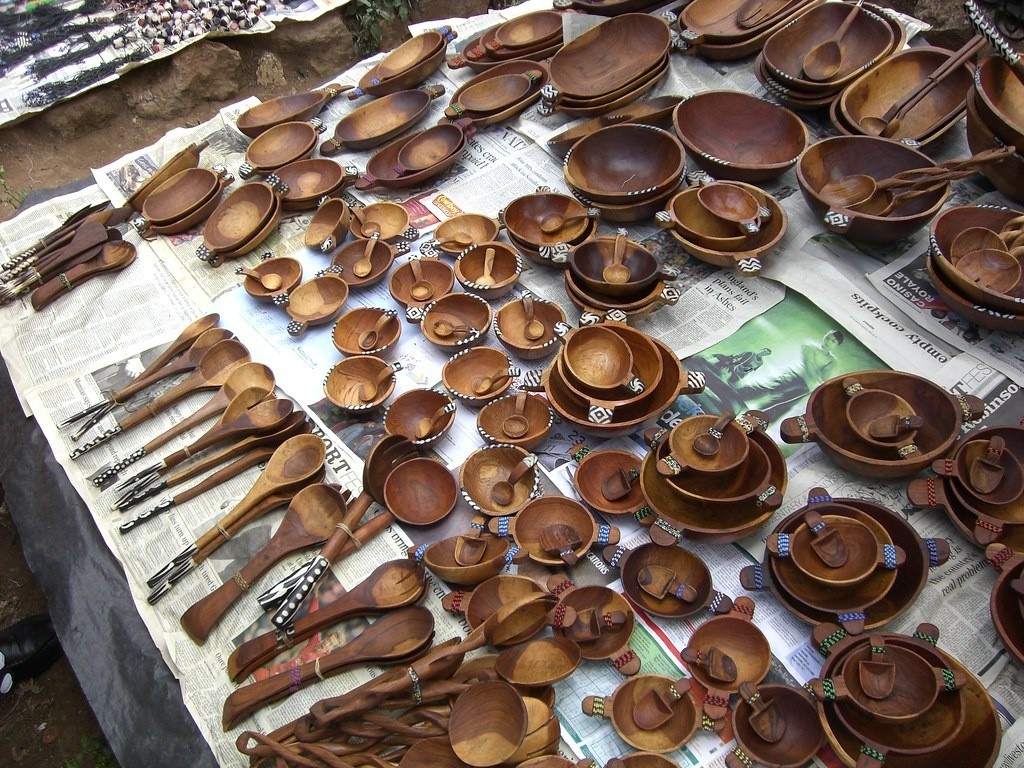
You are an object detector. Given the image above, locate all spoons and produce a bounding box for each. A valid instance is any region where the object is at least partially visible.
[0,203,435,729]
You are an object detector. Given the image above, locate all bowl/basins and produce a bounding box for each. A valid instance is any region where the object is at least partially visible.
[141,0,1024,768]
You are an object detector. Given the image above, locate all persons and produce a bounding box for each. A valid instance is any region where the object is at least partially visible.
[736,329,844,425]
[207,516,283,583]
[91,339,175,394]
[329,415,387,454]
[911,267,993,342]
[253,571,346,682]
[712,348,772,387]
